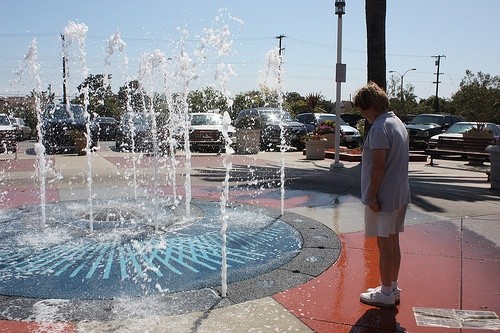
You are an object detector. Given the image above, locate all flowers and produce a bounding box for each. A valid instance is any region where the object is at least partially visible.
[303,132,324,140]
[315,120,336,133]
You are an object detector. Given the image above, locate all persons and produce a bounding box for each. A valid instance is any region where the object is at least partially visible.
[352,80,410,307]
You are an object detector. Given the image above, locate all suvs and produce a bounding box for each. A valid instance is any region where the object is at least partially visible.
[404,114,462,150]
[294,113,361,146]
[232,107,307,151]
[0,103,229,155]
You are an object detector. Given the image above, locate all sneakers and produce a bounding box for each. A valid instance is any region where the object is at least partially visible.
[359,285,402,309]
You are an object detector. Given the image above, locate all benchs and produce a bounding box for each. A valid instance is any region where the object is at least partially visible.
[426,136,497,165]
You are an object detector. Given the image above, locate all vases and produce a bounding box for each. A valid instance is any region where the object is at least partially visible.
[304,137,327,160]
[320,133,334,147]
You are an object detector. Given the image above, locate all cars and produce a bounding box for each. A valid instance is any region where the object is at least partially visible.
[428,122,500,153]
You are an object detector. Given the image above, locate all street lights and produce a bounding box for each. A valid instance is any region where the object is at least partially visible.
[389,68,416,102]
